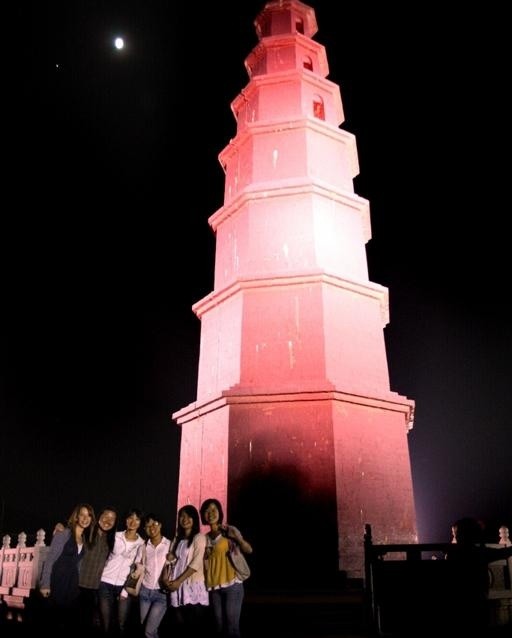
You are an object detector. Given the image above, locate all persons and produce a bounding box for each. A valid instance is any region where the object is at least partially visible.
[428,518,512,638]
[39,498,253,638]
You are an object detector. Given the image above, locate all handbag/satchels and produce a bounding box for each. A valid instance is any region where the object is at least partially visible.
[229,543,251,581]
